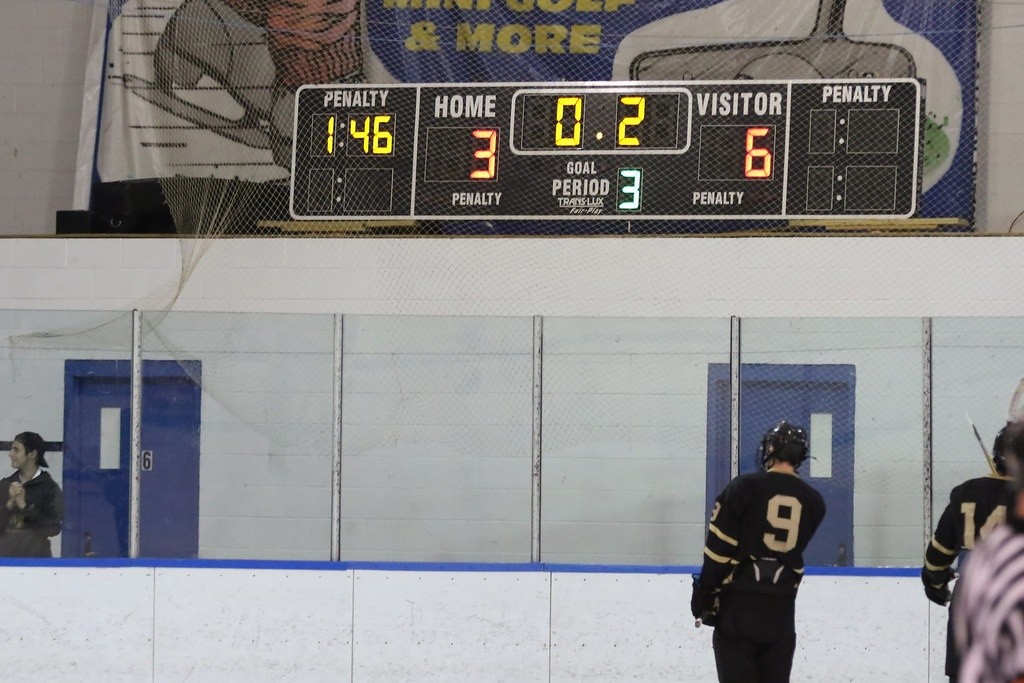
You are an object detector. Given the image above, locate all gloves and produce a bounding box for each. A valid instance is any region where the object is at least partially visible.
[925,583,951,606]
[690,572,722,627]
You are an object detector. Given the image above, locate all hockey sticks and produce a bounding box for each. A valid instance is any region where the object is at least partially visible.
[695,609,711,628]
[971,424,999,477]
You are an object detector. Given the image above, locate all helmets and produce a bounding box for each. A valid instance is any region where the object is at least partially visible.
[761,420,807,464]
[993,421,1024,476]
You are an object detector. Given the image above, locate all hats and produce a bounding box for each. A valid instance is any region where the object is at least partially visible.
[15,431,49,468]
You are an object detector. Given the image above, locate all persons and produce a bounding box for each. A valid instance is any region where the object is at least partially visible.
[0,432,64,559]
[691,421,827,683]
[921,423,1024,683]
